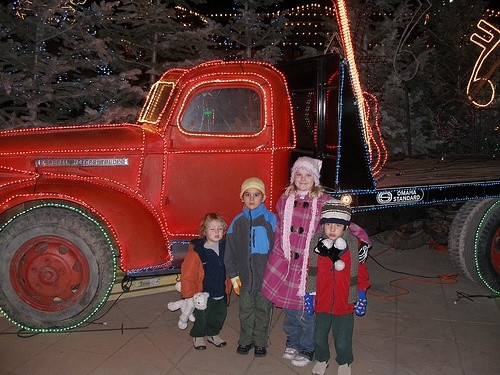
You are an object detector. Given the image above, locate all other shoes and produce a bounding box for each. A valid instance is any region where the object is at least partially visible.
[282,348,299,360]
[192,335,207,350]
[312,360,329,375]
[236,343,254,354]
[207,335,227,347]
[291,354,310,367]
[254,346,265,356]
[338,362,351,375]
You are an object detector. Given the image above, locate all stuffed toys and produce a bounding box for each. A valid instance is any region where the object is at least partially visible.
[168,292,210,329]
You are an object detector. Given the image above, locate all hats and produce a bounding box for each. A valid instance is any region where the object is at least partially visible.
[320,199,351,227]
[240,177,266,199]
[291,156,322,187]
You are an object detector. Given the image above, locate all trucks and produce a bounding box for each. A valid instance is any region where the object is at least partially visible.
[0,49,499,332]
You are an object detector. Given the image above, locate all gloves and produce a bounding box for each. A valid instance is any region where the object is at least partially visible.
[357,240,371,264]
[354,291,366,316]
[231,276,242,295]
[304,293,313,315]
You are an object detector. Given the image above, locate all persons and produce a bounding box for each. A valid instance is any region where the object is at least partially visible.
[224,177,276,356]
[179,213,232,349]
[261,157,373,367]
[305,199,371,375]
